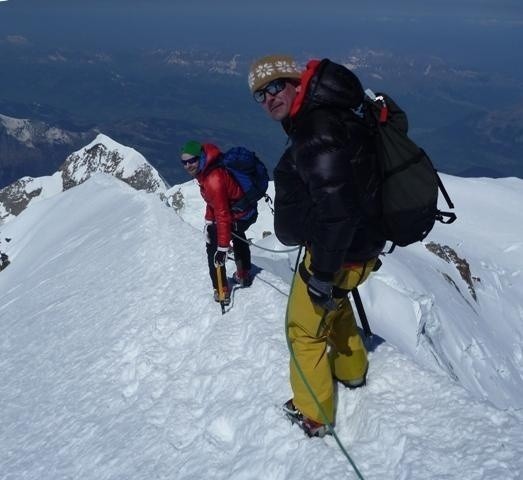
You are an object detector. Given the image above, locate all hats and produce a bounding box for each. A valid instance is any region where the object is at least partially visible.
[248,56,302,92]
[179,141,203,156]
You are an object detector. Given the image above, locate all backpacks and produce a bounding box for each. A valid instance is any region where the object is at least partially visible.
[354,91,438,247]
[204,143,269,214]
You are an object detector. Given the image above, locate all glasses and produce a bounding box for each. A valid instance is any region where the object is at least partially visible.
[181,156,200,166]
[253,78,286,103]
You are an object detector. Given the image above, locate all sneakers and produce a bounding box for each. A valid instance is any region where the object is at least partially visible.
[283,398,328,437]
[214,287,230,302]
[233,270,249,283]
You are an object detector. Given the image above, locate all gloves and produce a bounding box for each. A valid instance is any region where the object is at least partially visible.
[306,276,339,311]
[213,247,229,268]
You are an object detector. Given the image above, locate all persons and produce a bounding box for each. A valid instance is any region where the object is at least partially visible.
[178,140,258,305]
[249,55,387,438]
